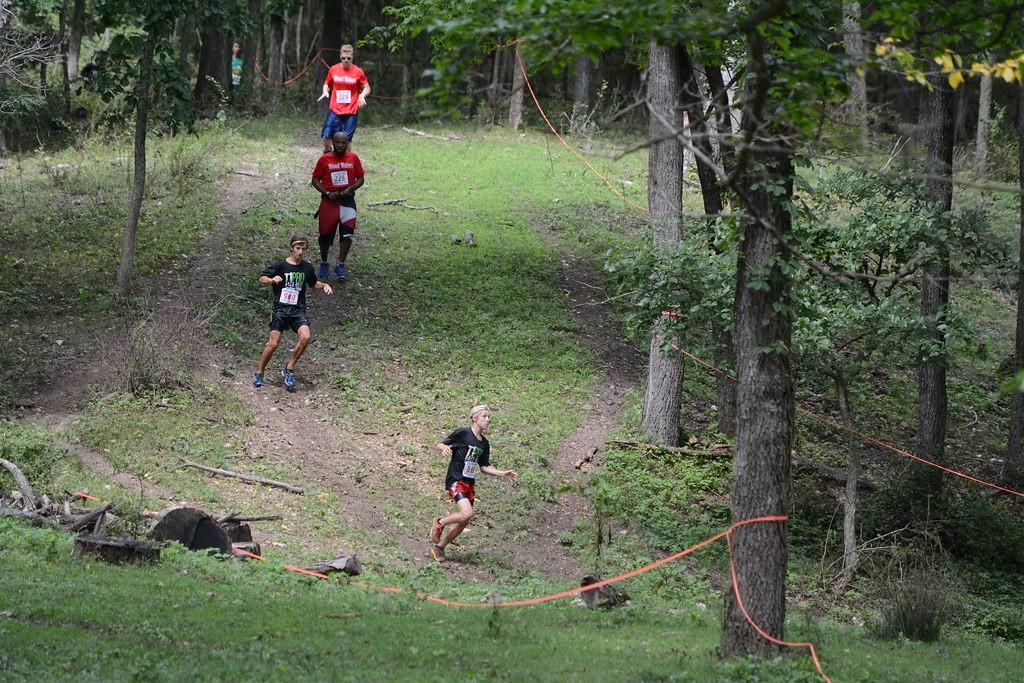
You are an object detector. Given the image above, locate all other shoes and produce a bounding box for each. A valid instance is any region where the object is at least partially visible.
[324,146,333,154]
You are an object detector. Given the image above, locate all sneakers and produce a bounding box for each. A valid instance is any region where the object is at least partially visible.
[282,366,295,387]
[431,543,447,563]
[252,372,263,387]
[430,517,443,542]
[335,262,345,280]
[320,263,329,279]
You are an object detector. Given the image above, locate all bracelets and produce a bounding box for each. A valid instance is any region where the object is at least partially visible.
[325,190,330,197]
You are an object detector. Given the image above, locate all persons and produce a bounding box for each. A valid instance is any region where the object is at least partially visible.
[252,235,333,388]
[312,132,365,281]
[231,38,243,91]
[317,44,371,155]
[430,405,518,562]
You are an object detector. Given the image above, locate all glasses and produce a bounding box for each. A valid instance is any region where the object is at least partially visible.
[341,57,352,60]
